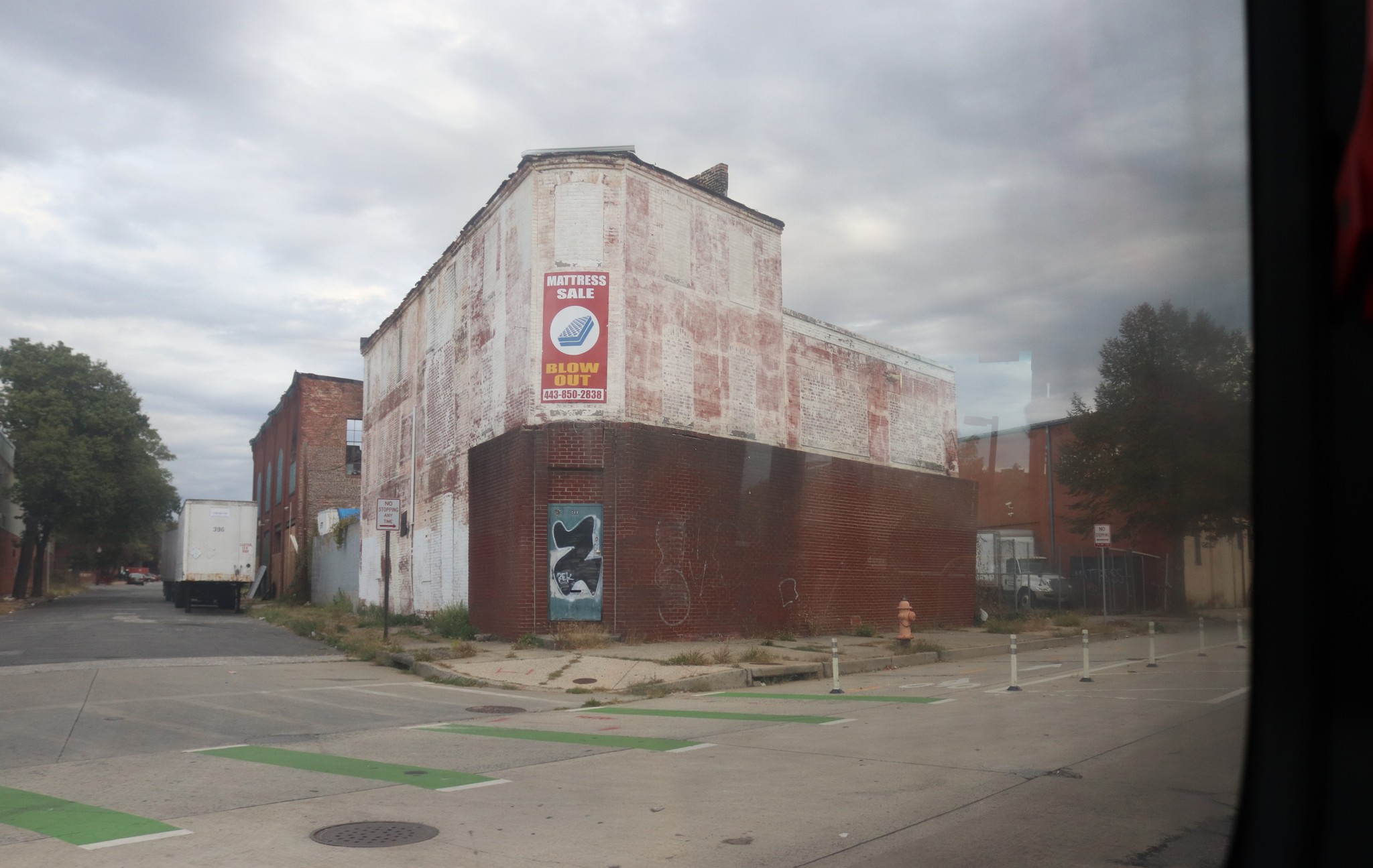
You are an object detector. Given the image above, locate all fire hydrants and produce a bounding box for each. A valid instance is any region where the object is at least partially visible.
[897,598,916,641]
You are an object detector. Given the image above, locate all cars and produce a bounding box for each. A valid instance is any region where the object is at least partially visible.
[127,573,144,585]
[143,575,157,582]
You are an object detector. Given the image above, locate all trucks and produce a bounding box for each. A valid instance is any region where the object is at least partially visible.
[976,530,1073,609]
[124,567,160,580]
[160,499,258,610]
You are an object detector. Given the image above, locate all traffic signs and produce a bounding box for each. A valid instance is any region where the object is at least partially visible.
[375,498,400,531]
[1094,524,1111,548]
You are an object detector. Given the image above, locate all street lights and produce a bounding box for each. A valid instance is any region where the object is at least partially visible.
[284,506,291,596]
